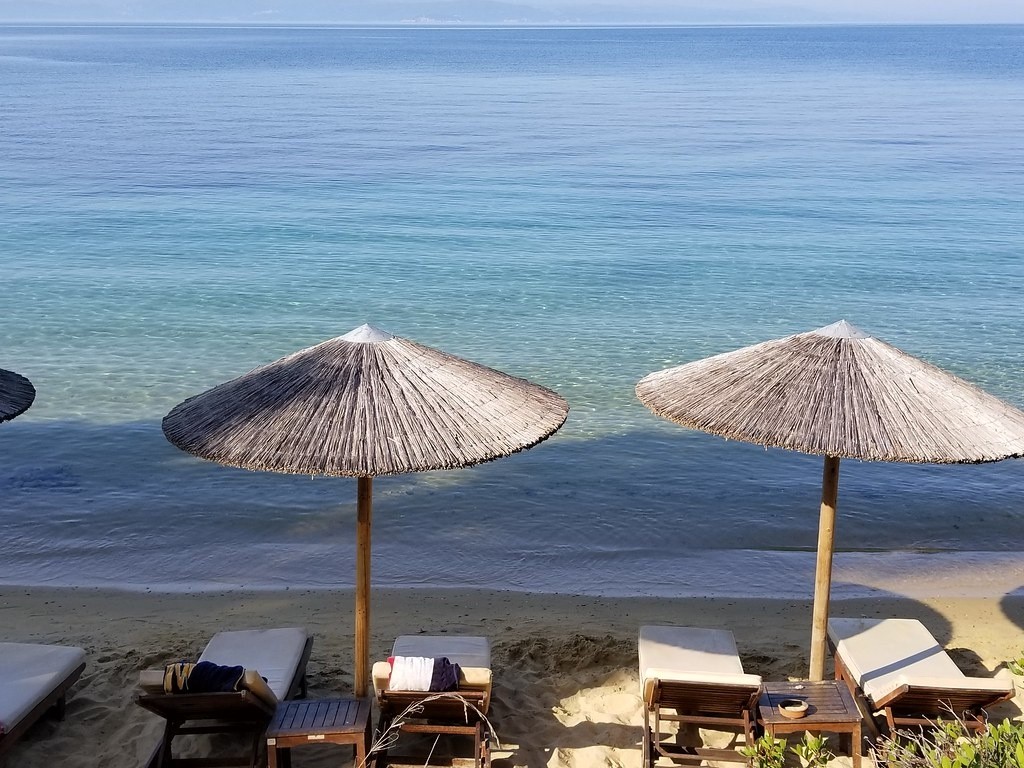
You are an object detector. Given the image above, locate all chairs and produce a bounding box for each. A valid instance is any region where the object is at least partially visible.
[828,615,1017,753]
[367,635,494,768]
[638,625,763,768]
[134,626,314,768]
[0,642,87,760]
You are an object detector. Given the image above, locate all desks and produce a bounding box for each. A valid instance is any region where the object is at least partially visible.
[265,696,372,768]
[758,679,863,768]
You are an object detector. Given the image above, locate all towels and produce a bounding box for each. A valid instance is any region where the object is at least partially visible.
[161,660,268,693]
[387,654,463,691]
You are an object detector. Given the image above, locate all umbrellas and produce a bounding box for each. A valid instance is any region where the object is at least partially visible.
[162,324,569,700]
[0,368,36,425]
[634,320,1024,682]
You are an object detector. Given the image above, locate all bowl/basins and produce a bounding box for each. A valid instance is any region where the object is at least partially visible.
[778,699,808,718]
